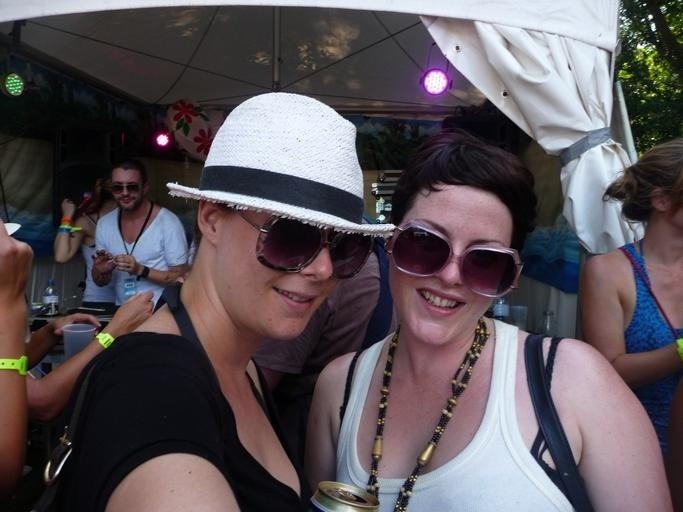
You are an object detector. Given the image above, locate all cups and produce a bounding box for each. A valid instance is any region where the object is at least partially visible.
[60,323,98,362]
[510,304,530,331]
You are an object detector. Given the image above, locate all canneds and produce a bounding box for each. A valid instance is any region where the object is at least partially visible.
[308,481,380,512]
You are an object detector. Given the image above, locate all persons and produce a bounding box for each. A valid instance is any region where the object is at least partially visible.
[91,158,190,315]
[575,136,683,455]
[52,168,118,311]
[0,217,34,509]
[301,127,674,511]
[253,245,381,411]
[25,289,155,422]
[364,209,393,349]
[52,90,396,510]
[663,375,683,512]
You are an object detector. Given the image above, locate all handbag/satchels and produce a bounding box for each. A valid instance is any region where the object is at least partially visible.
[0,444,76,510]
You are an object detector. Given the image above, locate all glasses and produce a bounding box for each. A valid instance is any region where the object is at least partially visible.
[385,220,522,299]
[238,211,373,280]
[110,182,141,193]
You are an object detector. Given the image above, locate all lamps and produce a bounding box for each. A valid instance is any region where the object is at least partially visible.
[420,42,453,96]
[1,54,25,99]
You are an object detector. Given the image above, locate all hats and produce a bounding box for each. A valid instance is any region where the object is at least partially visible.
[167,92,398,239]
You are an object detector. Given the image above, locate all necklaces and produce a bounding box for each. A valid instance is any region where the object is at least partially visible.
[367,312,490,511]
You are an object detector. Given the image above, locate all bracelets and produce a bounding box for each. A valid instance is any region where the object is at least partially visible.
[96,331,114,348]
[675,335,683,357]
[57,215,82,235]
[0,354,30,376]
[136,264,151,283]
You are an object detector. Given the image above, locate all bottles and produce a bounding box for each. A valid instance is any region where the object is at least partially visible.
[540,310,556,336]
[41,278,61,317]
[491,297,512,324]
